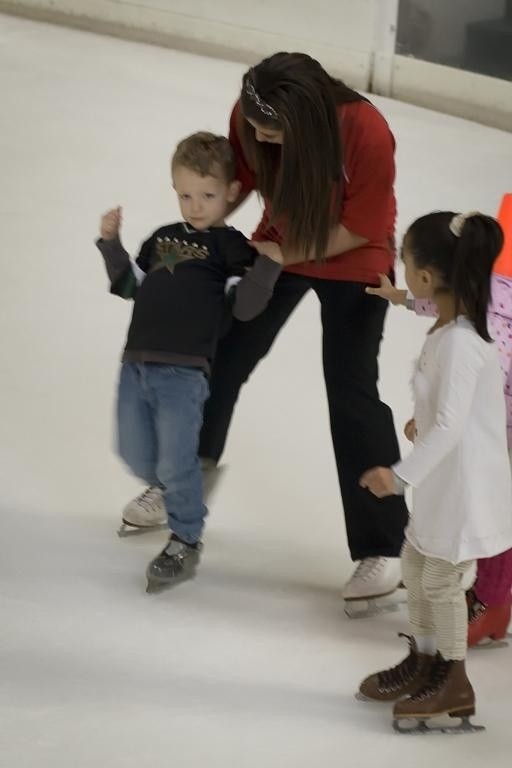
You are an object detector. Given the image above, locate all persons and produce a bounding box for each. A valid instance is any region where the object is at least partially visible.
[121,52,409,618]
[359,210,512,734]
[95,132,282,582]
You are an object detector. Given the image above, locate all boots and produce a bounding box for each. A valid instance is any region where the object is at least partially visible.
[146,534,203,582]
[466,584,511,648]
[341,554,403,599]
[121,484,170,527]
[391,655,476,719]
[359,649,433,701]
